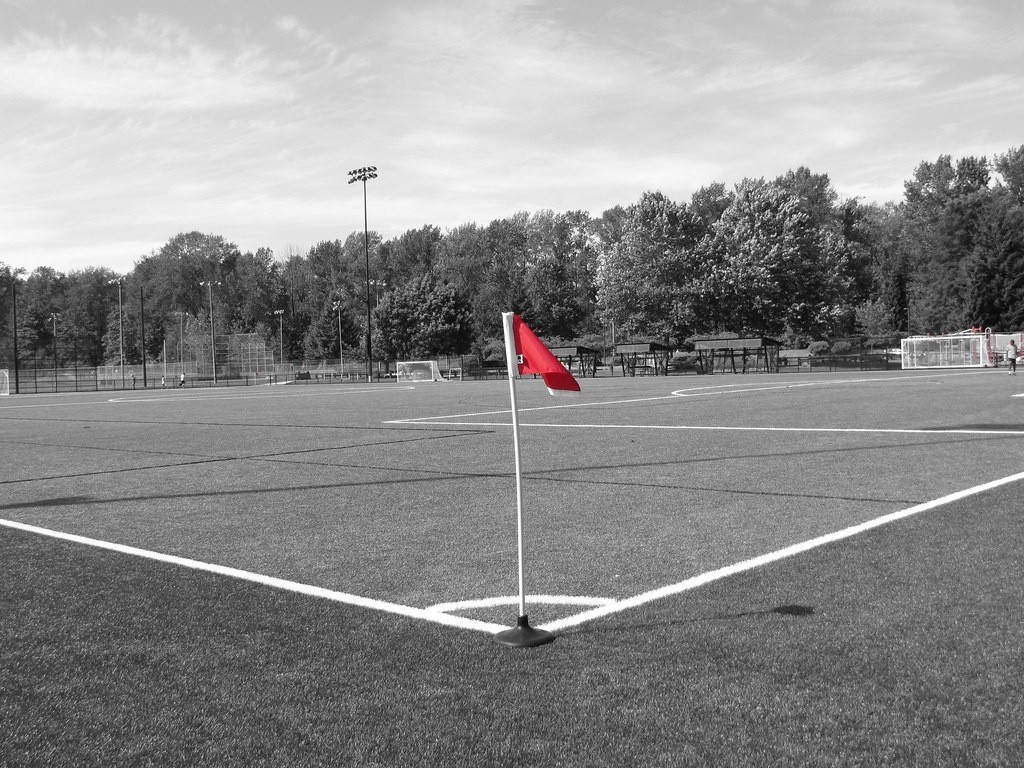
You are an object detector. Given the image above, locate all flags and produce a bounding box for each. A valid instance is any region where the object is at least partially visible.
[502,312,582,398]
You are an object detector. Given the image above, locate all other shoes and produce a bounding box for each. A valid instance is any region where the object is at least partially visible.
[1009,370,1011,375]
[1013,373,1016,375]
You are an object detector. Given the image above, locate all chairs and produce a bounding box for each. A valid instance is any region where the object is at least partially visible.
[630,366,654,377]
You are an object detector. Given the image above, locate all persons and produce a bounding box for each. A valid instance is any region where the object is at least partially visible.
[161,375,165,389]
[1004,340,1017,375]
[179,373,185,389]
[131,374,135,390]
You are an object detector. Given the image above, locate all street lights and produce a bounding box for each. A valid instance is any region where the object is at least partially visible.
[12,280,23,395]
[107,276,128,390]
[331,301,345,382]
[199,281,222,384]
[347,165,378,383]
[47,312,62,392]
